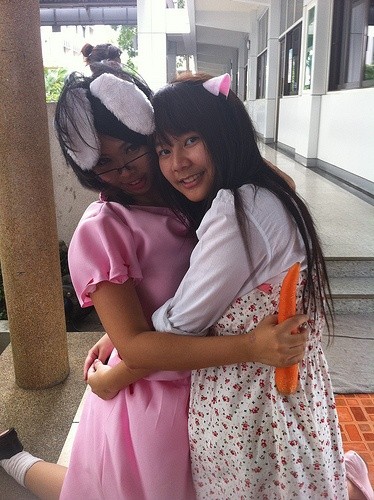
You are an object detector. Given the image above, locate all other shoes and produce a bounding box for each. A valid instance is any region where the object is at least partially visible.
[0,427,24,460]
[344,450,374,500]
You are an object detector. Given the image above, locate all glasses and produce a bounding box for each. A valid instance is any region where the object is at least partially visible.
[94,151,153,183]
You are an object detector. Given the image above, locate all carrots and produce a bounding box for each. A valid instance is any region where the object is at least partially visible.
[275,261,302,395]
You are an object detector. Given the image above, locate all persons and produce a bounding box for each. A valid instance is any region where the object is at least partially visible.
[0,44,374,500]
